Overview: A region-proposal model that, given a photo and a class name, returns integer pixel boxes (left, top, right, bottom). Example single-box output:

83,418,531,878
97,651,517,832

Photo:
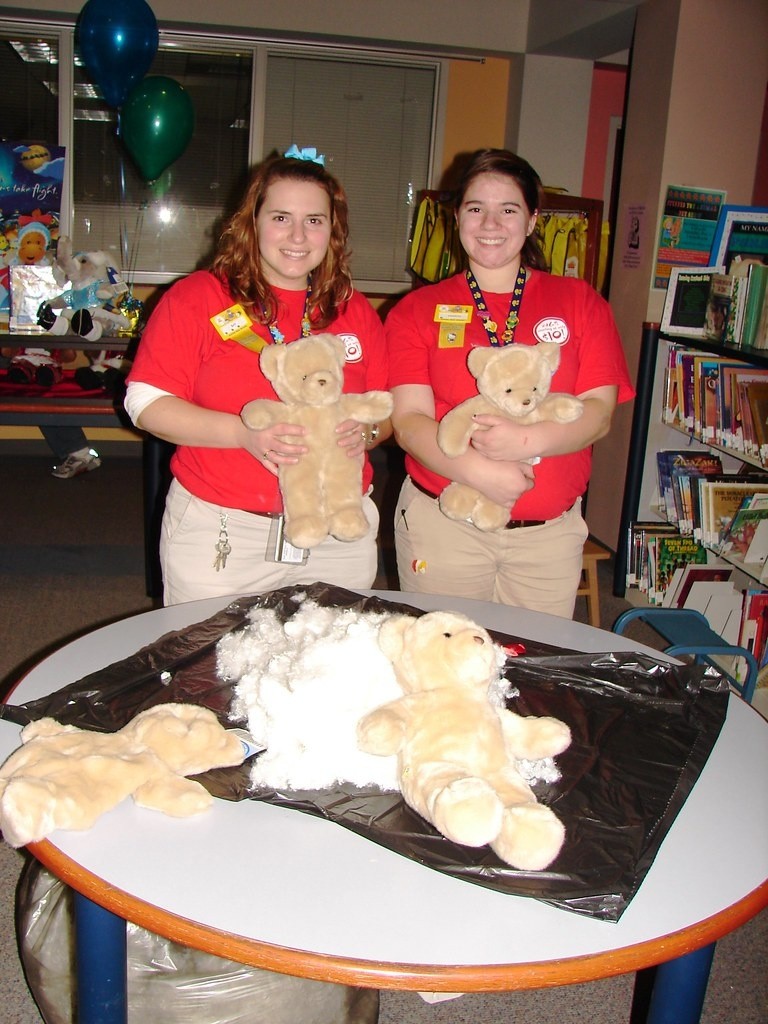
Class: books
650,185,768,355
657,447,767,586
660,343,768,468
624,521,768,692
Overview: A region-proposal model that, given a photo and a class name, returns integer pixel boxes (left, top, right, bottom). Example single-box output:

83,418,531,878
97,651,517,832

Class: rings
362,432,366,440
264,450,275,460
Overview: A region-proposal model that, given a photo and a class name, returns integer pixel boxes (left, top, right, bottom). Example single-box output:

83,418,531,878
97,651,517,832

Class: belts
410,474,577,531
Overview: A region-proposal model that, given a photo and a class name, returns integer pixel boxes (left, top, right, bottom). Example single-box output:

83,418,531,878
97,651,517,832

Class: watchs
372,423,380,442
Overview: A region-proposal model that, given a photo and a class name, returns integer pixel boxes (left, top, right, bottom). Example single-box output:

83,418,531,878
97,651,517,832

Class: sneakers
51,448,101,478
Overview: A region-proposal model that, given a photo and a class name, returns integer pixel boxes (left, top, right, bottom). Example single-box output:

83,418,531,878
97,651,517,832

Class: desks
0,587,767,1021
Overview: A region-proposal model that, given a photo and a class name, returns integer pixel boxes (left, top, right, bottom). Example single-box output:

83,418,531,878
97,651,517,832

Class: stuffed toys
240,329,370,547
358,610,572,872
435,340,584,532
42,232,131,342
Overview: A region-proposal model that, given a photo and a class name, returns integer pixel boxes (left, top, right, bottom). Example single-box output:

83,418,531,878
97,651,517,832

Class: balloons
73,0,159,110
123,76,193,184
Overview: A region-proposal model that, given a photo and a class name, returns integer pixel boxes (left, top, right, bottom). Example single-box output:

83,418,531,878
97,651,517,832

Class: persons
41,424,102,478
121,144,394,615
384,147,638,621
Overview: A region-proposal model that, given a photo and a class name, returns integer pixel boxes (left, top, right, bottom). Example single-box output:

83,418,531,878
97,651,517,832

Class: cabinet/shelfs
609,320,767,719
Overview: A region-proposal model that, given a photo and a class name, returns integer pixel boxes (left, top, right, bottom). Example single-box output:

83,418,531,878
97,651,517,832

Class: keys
212,542,232,573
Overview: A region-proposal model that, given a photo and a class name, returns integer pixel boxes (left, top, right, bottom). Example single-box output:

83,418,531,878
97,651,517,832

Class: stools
612,607,756,704
572,536,611,629
